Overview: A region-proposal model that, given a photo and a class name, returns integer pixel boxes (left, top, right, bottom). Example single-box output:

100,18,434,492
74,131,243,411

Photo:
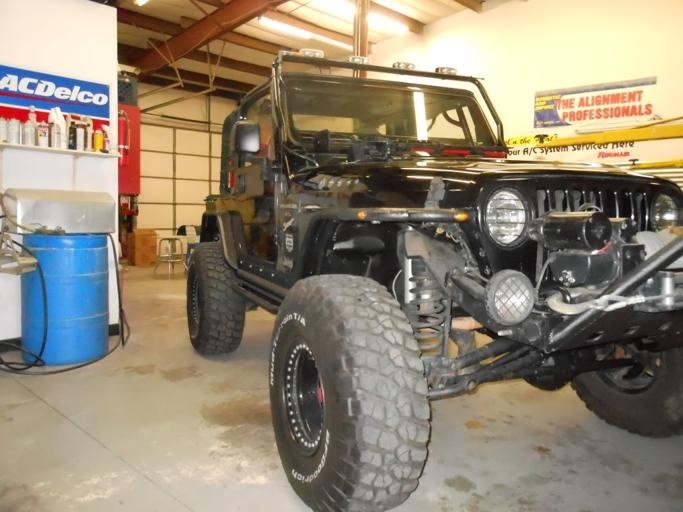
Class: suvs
186,46,683,512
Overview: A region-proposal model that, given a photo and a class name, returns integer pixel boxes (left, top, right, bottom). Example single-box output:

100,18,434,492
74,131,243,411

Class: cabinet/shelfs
0,96,122,160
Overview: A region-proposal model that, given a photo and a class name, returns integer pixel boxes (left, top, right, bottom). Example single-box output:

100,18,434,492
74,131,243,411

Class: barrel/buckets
20,233,110,365
48,107,67,150
